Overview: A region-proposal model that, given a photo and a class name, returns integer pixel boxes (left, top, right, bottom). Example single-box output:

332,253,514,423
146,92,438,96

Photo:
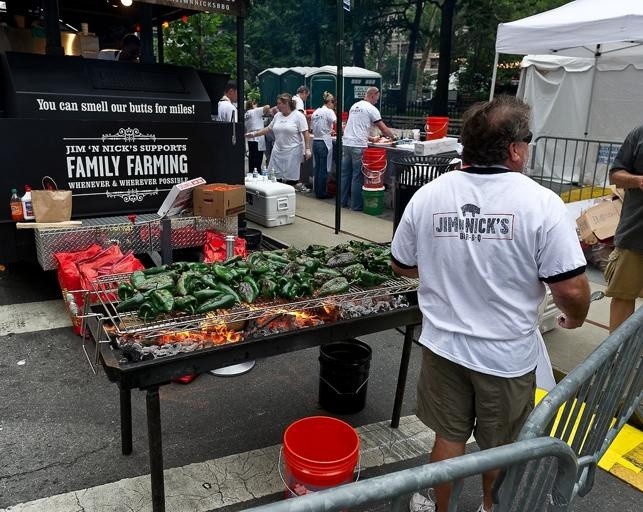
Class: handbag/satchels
30,174,74,224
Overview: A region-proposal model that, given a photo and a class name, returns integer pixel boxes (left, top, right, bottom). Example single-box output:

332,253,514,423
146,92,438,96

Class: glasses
517,130,532,144
375,89,380,94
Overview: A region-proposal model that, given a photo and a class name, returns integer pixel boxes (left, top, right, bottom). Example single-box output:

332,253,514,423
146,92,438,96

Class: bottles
252,166,276,182
9,188,23,221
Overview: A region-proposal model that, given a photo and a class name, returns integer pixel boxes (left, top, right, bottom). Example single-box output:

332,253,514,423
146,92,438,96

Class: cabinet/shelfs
79,294,421,511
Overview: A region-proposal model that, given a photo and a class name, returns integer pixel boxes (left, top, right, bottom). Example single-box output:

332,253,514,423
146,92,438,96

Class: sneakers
409,491,437,512
476,502,493,512
294,183,310,193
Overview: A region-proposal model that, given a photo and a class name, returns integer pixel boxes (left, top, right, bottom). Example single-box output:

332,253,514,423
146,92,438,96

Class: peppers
117,237,393,318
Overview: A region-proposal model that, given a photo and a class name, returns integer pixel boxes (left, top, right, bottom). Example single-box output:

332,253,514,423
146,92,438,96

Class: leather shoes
319,194,333,199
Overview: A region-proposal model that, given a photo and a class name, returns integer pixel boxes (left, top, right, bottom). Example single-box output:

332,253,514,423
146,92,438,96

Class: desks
329,132,459,189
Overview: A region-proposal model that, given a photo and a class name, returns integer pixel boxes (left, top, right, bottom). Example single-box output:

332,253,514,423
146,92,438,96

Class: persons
340,85,394,212
602,124,642,335
388,90,591,512
216,82,340,199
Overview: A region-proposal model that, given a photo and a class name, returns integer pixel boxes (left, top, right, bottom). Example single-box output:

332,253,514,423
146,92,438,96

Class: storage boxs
192,182,246,219
244,178,295,228
412,137,457,156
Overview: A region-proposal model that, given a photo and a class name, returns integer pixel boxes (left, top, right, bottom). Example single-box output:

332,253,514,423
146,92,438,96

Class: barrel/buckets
425,117,450,140
317,339,373,414
361,148,387,216
306,109,316,132
276,416,363,500
332,111,347,136
